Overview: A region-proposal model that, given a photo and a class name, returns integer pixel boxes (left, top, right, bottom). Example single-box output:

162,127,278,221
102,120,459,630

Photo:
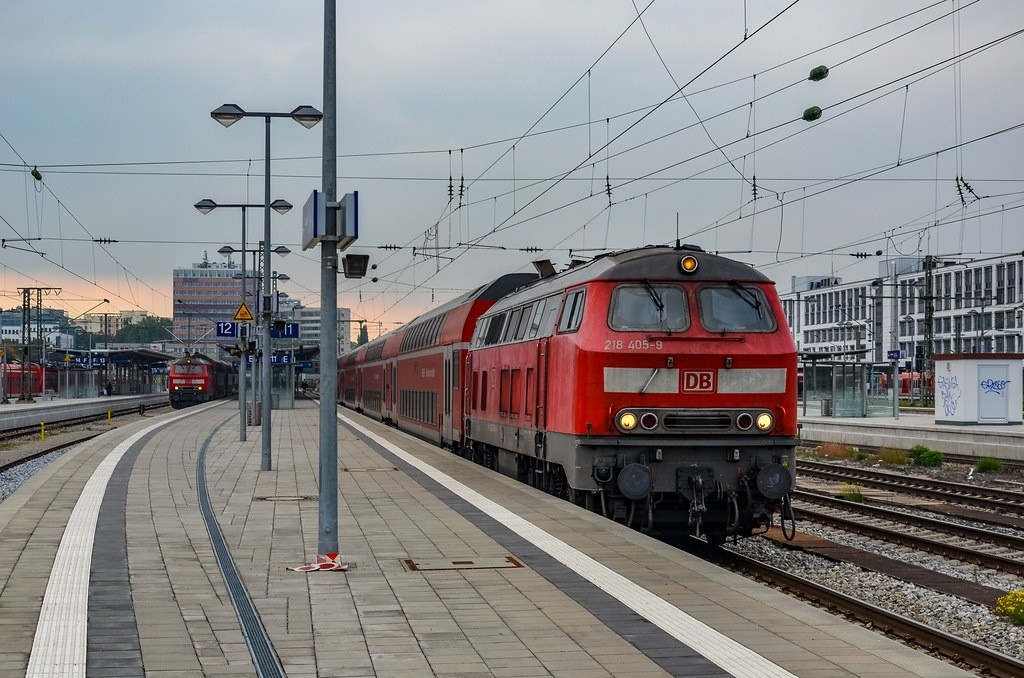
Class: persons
104,381,114,399
300,379,309,393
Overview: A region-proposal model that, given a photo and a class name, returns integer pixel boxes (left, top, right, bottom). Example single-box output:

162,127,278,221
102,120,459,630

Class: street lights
194,199,295,442
210,99,323,473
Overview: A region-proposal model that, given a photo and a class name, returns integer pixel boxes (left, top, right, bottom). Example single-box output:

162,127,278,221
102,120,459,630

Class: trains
338,237,800,548
168,350,240,409
0,362,58,396
